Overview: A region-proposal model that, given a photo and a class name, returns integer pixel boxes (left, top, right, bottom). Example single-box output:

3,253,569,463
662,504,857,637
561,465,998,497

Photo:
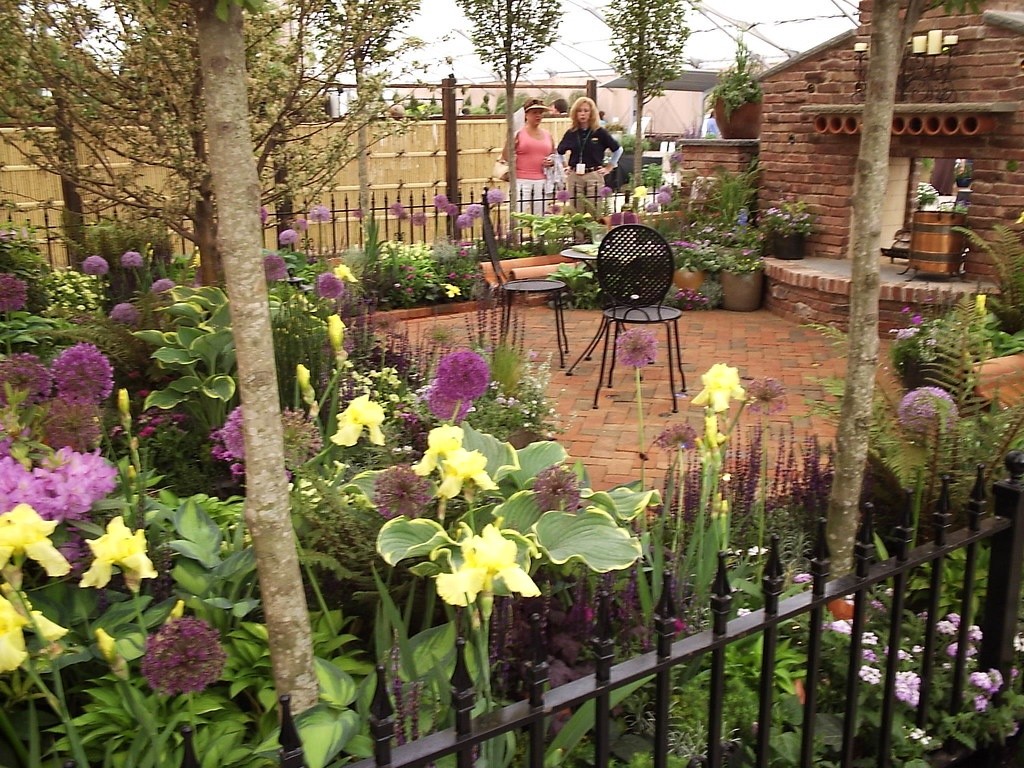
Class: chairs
593,223,688,413
481,201,570,369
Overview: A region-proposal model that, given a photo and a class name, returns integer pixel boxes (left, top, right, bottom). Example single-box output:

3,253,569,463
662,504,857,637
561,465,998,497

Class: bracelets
563,166,570,171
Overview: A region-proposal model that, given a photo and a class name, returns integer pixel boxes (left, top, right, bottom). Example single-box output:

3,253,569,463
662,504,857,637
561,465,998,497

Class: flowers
664,193,827,313
703,27,760,122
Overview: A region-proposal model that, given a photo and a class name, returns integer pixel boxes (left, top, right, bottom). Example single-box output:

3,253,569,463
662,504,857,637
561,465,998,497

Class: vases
673,265,708,294
715,97,759,140
719,270,760,313
956,177,971,187
774,230,807,259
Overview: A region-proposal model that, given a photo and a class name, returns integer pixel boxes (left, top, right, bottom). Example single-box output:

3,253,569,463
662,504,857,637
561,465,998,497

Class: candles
927,29,944,55
945,35,958,44
855,42,868,50
912,36,928,53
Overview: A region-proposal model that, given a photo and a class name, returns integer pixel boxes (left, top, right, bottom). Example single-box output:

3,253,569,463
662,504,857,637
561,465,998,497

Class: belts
569,165,603,174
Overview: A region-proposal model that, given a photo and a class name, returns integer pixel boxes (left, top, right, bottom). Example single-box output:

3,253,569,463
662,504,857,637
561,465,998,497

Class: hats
524,99,553,113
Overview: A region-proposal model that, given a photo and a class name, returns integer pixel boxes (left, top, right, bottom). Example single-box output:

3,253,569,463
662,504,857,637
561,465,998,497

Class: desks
561,245,659,383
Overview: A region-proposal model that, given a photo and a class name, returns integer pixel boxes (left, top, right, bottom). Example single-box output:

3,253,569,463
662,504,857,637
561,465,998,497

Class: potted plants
613,134,662,186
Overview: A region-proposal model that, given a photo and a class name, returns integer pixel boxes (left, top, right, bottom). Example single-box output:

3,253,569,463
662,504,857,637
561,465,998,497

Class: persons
548,98,568,114
389,105,405,121
706,109,721,139
598,110,606,126
555,97,624,244
498,97,556,234
